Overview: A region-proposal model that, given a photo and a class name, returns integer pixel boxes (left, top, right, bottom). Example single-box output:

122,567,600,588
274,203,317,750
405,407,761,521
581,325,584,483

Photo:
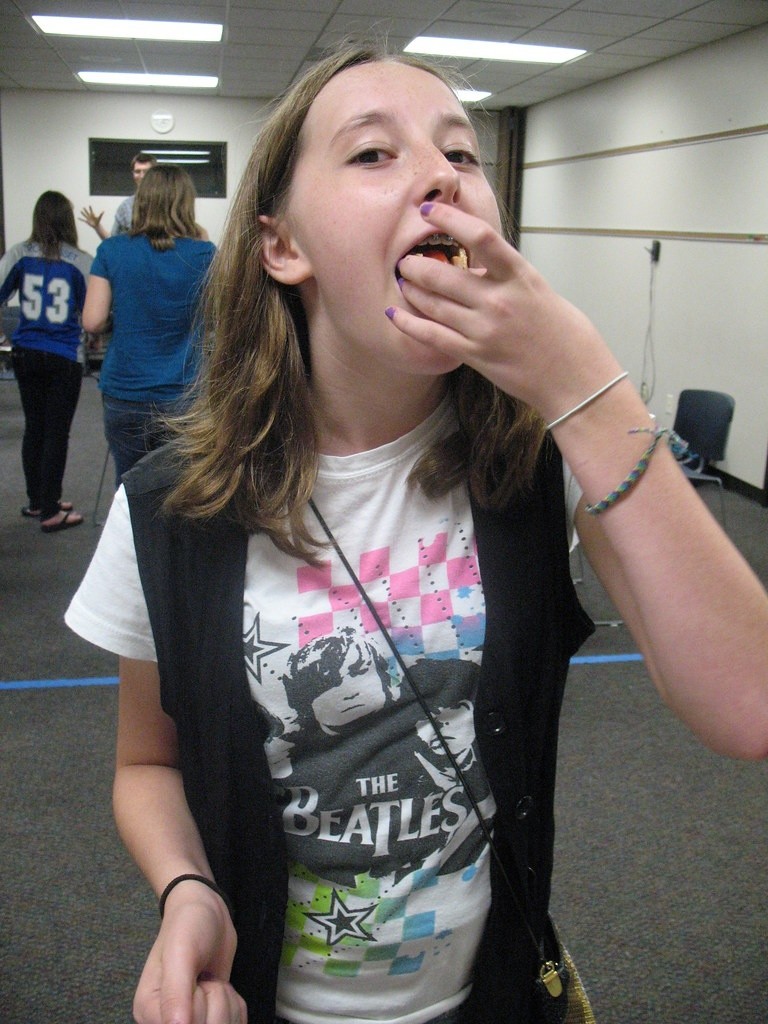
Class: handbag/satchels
534,914,592,1024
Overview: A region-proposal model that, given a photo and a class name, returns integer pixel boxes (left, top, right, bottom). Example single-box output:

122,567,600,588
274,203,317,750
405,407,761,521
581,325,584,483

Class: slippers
21,502,72,516
41,513,83,531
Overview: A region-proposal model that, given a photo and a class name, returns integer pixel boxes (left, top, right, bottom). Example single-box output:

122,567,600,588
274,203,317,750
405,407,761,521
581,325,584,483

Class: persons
59,41,767,1024
0,153,219,529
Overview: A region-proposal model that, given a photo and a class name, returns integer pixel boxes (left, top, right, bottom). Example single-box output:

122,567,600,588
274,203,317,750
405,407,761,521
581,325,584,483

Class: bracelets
158,873,230,919
543,370,628,428
585,423,689,515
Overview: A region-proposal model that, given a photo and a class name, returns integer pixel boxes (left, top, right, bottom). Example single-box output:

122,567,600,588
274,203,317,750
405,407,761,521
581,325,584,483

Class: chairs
672,389,735,524
560,456,624,626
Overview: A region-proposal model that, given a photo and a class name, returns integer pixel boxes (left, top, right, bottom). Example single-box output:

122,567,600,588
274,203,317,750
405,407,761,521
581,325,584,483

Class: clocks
151,109,175,134
